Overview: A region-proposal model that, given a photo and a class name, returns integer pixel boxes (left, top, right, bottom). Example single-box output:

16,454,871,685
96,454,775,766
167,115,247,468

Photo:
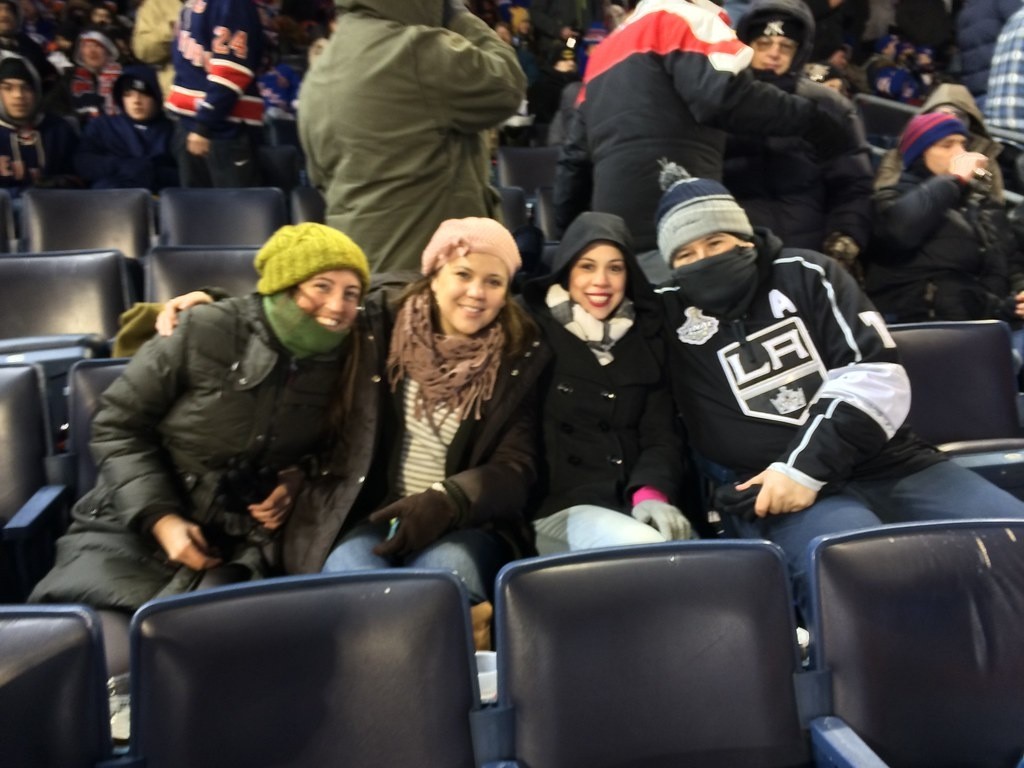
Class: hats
73,20,119,69
735,8,805,52
112,71,161,116
507,6,534,38
0,58,36,95
898,114,973,171
254,221,372,297
652,157,758,268
420,218,523,285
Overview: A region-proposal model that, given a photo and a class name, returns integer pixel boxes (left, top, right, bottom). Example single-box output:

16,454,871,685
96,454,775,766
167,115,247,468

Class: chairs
1,146,1024,768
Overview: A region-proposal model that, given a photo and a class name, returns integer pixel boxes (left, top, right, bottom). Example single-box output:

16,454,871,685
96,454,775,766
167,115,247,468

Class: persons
29,224,369,687
649,161,1024,655
537,207,697,557
468,0,544,144
862,0,1024,322
154,217,536,652
295,0,528,274
0,0,346,191
547,0,863,260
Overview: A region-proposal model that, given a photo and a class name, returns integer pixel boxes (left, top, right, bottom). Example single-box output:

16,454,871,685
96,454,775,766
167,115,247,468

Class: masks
672,245,758,304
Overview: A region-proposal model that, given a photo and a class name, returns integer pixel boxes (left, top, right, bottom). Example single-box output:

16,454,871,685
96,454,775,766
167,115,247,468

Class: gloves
367,490,459,569
633,487,693,543
